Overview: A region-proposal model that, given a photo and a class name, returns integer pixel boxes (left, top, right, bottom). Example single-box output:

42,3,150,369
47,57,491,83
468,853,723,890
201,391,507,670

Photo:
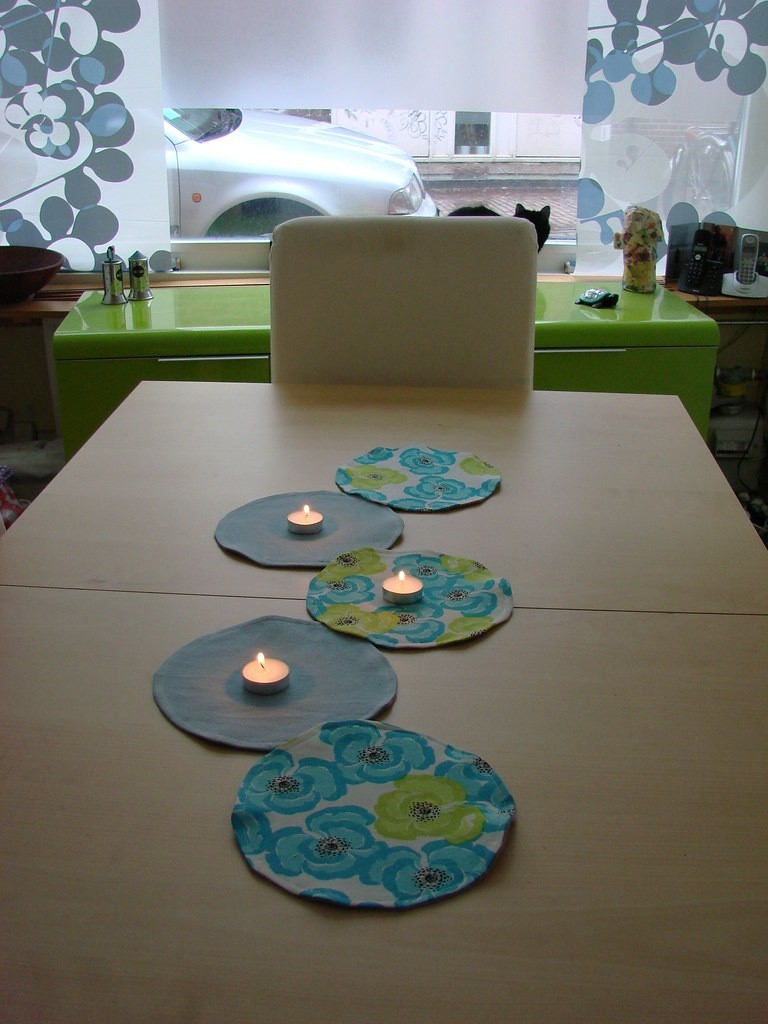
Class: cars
163,107,440,240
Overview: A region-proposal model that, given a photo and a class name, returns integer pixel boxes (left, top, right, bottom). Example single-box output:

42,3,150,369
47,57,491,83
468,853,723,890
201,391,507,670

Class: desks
1,380,768,1024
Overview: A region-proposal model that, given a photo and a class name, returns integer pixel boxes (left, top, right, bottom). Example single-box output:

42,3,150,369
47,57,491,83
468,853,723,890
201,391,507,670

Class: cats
447,204,551,253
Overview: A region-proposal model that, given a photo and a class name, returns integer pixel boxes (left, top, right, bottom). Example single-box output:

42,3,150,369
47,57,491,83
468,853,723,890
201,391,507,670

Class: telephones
722,234,768,298
677,229,725,295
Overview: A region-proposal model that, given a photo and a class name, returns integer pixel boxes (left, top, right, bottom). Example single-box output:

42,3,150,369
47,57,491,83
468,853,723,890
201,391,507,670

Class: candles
383,574,423,603
286,510,323,534
241,658,290,694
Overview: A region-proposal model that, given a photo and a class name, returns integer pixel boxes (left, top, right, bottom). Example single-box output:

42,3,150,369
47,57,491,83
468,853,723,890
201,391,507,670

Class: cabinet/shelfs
53,281,717,443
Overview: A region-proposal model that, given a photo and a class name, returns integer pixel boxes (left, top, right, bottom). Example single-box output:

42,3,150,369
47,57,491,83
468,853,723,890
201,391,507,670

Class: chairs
268,215,536,390
